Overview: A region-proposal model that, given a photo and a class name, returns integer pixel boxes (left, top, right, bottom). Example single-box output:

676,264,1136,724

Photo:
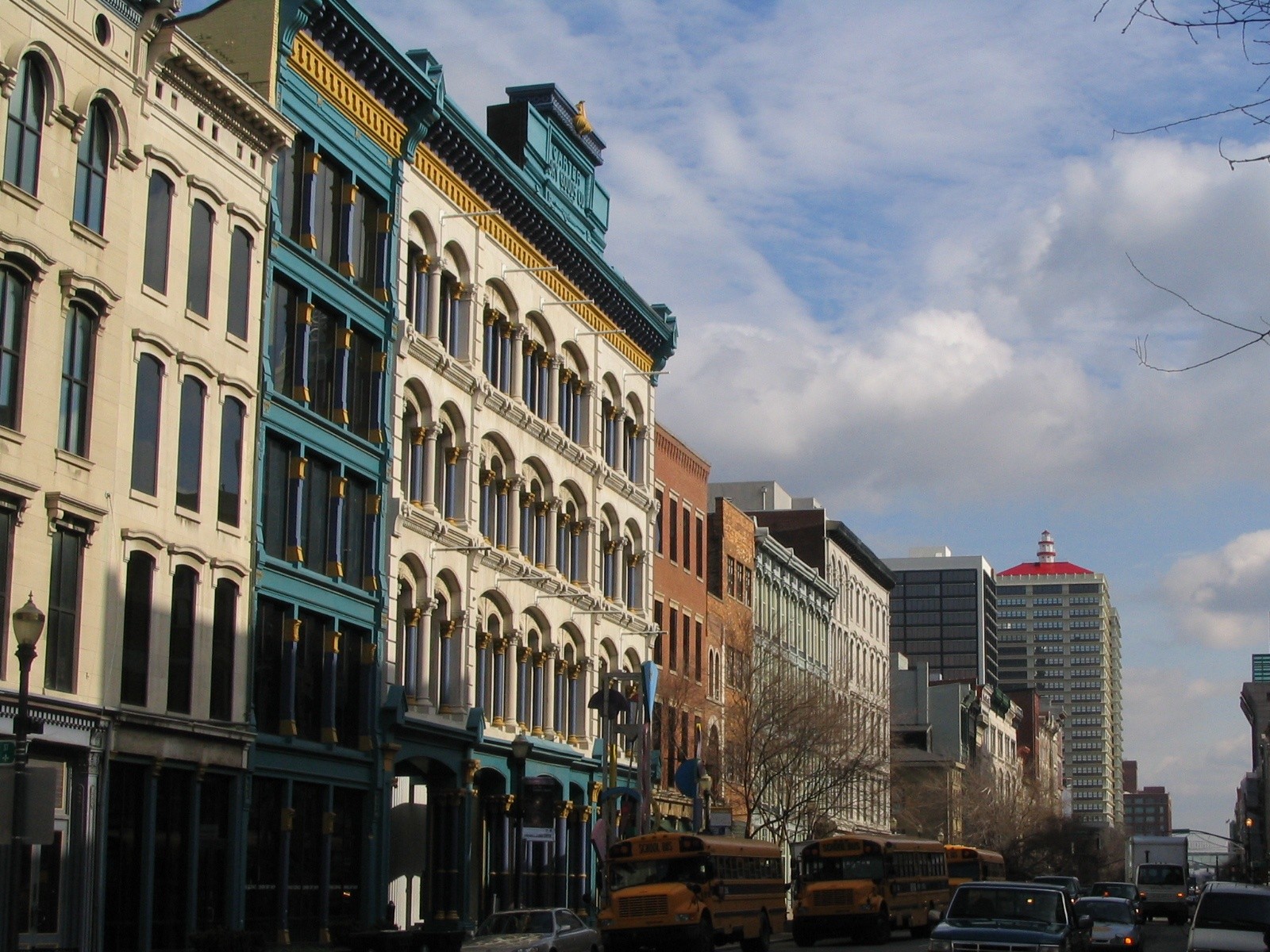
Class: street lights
4,597,46,952
696,774,713,831
509,735,531,916
1169,828,1248,881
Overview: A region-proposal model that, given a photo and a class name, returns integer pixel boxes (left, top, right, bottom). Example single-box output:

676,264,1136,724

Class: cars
1033,874,1088,907
1084,880,1146,919
1074,896,1144,952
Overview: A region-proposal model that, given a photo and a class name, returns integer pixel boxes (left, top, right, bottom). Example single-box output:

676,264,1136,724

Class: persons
1165,868,1180,885
1118,887,1130,898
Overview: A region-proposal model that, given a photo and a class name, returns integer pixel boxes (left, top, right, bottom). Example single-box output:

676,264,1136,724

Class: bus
595,831,786,951
791,833,950,945
944,846,1007,893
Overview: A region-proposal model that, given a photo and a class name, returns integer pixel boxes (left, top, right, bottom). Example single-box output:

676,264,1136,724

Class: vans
1188,881,1270,952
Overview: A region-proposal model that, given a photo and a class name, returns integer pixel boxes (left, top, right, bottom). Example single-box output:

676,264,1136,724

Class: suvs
927,880,1095,951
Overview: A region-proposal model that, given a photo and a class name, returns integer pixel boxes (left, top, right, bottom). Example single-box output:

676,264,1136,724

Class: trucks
1128,833,1199,924
